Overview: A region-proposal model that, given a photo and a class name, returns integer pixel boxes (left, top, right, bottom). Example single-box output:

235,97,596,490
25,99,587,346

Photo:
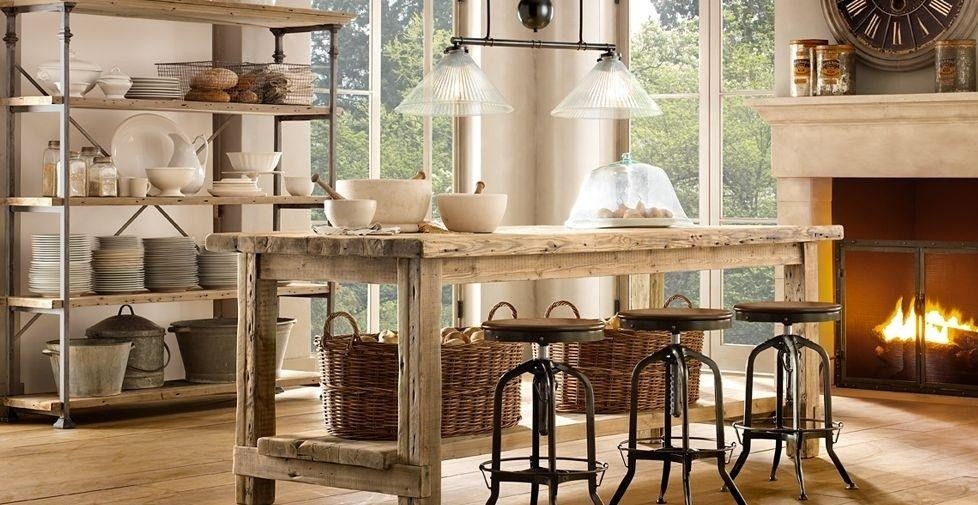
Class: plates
127,76,183,99
208,178,268,199
27,232,239,298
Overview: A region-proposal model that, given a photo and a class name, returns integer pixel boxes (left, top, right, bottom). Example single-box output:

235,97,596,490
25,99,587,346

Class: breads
184,67,258,102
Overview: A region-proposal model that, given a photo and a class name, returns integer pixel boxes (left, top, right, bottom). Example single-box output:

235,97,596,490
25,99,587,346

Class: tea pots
168,131,209,194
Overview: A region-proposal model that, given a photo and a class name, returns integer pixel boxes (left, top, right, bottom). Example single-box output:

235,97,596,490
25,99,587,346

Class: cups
120,176,151,197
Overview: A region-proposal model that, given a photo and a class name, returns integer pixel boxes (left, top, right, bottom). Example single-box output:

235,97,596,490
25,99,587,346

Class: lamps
393,0,663,120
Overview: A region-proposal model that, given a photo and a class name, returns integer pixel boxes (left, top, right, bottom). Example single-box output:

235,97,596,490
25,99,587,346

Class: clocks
814,1,977,72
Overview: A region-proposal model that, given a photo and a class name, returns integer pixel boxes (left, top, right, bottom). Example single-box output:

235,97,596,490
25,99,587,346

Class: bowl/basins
37,48,133,99
225,149,282,171
145,168,197,197
112,113,193,170
284,175,314,195
324,178,508,233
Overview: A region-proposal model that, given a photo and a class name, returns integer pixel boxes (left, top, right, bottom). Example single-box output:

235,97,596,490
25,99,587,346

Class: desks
203,223,847,505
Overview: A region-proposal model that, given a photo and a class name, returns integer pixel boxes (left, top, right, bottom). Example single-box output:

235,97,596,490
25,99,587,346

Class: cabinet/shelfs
0,0,358,430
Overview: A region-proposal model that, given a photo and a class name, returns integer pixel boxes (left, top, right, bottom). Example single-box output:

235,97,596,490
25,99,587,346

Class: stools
609,307,747,505
480,317,608,505
722,302,859,501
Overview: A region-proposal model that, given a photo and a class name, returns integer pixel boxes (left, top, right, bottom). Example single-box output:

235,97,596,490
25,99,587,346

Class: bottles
43,139,118,197
788,38,854,97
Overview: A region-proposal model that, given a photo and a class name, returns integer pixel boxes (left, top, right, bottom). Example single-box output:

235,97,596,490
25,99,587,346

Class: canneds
41,141,118,197
788,39,856,97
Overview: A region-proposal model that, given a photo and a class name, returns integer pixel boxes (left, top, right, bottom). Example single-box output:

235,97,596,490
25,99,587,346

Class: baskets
154,62,314,106
313,301,524,440
530,294,704,414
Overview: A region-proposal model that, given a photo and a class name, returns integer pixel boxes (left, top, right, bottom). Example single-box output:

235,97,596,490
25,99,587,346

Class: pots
42,304,298,399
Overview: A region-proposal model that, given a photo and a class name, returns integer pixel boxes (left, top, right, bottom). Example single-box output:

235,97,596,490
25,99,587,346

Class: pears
355,328,484,349
609,314,621,329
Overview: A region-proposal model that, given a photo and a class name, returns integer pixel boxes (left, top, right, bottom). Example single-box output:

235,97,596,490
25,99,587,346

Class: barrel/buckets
86,306,172,388
38,336,134,397
